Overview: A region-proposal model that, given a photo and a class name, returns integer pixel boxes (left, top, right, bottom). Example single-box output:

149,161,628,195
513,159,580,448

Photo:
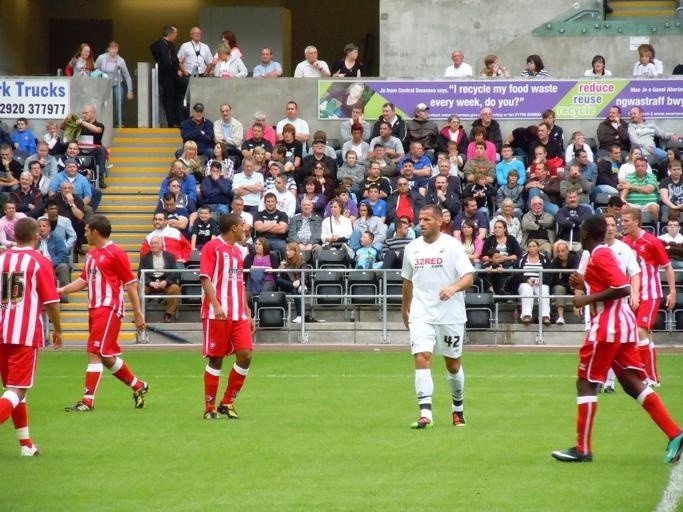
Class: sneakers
663,430,683,464
549,445,593,464
217,403,239,419
450,410,466,428
19,444,45,457
63,400,95,412
131,380,148,408
410,414,433,428
202,403,217,418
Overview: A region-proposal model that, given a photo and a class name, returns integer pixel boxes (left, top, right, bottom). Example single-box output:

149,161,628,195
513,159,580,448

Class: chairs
0,121,103,301
155,138,682,304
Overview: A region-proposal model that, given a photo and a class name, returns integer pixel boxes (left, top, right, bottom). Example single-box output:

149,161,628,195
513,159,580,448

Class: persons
213,30,248,78
295,46,332,78
633,44,664,77
619,206,676,389
521,55,549,77
1,103,107,303
199,214,254,419
332,43,362,78
94,41,133,129
480,55,509,76
150,25,185,127
584,56,611,77
399,202,476,430
445,50,472,76
54,214,148,412
572,215,642,395
177,27,213,83
550,214,682,463
139,102,683,324
0,218,63,455
253,48,283,78
65,43,94,77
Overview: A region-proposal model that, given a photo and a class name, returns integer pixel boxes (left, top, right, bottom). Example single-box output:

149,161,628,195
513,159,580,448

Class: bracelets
319,67,323,71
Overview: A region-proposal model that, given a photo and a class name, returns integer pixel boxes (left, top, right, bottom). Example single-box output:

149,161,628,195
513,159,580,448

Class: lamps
254,289,288,331
464,292,494,328
